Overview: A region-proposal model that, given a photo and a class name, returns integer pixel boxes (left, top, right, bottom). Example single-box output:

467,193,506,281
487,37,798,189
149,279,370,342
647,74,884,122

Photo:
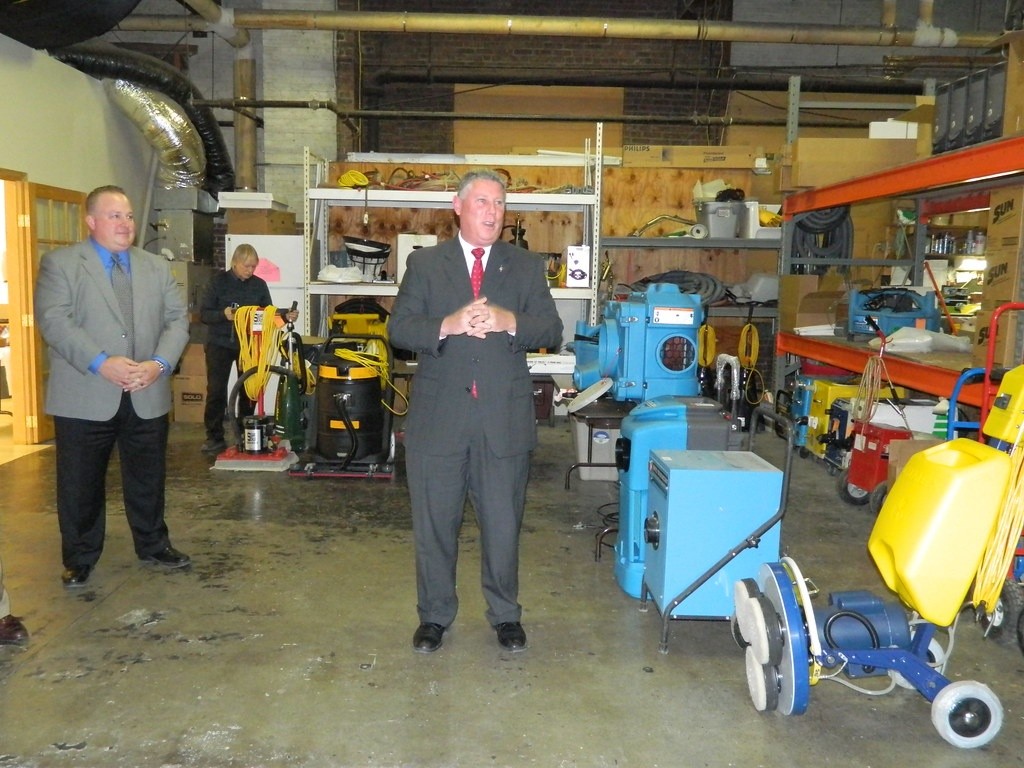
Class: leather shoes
497,621,527,651
62,557,93,586
138,546,190,567
412,622,446,653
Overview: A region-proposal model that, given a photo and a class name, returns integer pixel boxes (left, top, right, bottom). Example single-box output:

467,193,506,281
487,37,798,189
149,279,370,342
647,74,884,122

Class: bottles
924,229,987,255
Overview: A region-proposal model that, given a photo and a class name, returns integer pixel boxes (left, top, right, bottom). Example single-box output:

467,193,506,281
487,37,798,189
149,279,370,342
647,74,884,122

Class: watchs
156,360,164,373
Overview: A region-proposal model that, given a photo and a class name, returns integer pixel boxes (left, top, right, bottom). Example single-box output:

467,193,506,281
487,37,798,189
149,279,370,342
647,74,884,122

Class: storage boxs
983,30,1024,136
526,350,576,374
971,183,1024,365
849,398,958,442
695,201,743,239
569,414,622,481
562,245,590,288
781,274,847,334
218,192,289,212
396,231,438,283
887,439,945,494
226,208,297,235
173,343,207,423
773,104,937,193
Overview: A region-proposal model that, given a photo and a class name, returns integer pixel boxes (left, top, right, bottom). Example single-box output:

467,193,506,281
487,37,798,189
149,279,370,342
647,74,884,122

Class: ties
471,248,484,398
110,251,134,360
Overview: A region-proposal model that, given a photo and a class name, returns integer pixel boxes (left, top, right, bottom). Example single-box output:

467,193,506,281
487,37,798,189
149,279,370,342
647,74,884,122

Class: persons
388,169,565,653
199,244,299,451
36,185,192,586
0,559,29,644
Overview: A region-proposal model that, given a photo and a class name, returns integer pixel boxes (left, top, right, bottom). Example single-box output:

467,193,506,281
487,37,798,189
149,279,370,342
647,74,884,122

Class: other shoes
200,440,225,450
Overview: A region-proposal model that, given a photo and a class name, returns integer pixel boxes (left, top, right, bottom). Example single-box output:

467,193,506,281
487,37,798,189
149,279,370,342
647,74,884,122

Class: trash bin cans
568,376,637,482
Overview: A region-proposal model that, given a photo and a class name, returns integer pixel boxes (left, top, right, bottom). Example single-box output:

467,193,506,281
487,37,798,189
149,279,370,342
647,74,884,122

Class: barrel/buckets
243,415,269,455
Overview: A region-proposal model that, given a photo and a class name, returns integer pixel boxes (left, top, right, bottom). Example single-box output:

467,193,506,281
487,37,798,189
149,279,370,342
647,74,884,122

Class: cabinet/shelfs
304,121,604,382
598,236,780,318
884,223,988,321
767,74,1024,437
